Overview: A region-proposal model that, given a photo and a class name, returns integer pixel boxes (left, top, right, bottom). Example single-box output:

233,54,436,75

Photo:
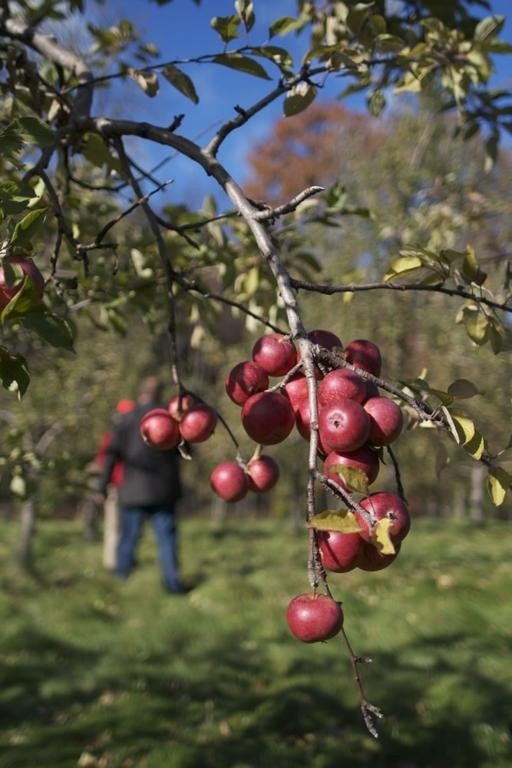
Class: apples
137,330,410,642
0,256,44,311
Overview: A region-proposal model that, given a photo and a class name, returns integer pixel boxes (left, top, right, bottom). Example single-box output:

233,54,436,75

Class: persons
88,375,189,593
96,397,141,572
